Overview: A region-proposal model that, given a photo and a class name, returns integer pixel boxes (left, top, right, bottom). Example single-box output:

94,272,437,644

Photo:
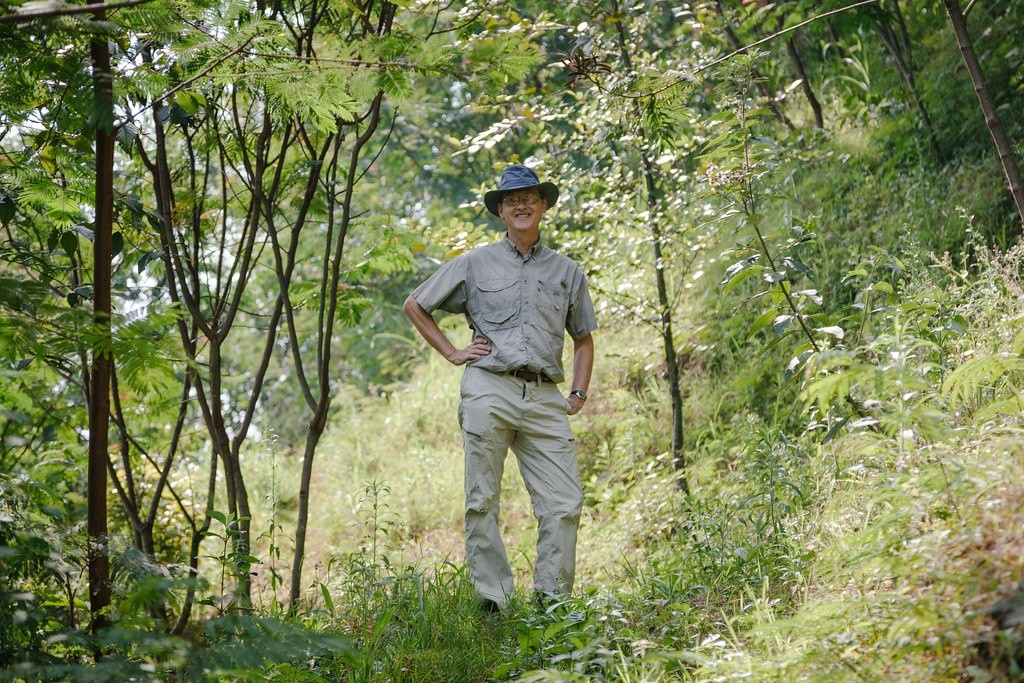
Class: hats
484,165,559,217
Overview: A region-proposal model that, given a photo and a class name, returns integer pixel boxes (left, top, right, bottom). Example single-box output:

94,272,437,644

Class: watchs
571,389,587,401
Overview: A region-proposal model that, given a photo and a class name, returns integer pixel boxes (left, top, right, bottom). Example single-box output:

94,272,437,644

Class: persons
402,165,597,615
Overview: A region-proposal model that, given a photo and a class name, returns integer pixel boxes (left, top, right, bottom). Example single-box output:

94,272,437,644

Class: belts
509,369,550,381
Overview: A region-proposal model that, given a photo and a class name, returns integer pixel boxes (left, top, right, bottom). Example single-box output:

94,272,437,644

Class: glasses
501,194,542,206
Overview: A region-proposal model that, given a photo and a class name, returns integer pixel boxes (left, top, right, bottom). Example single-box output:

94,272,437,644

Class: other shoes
479,601,501,616
